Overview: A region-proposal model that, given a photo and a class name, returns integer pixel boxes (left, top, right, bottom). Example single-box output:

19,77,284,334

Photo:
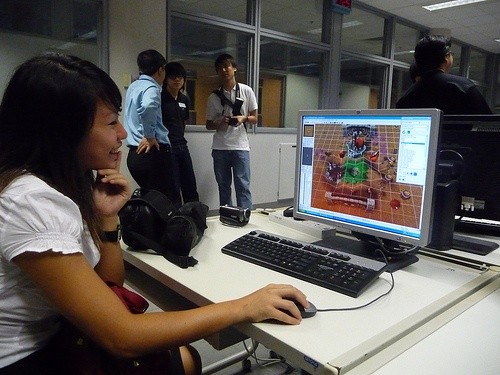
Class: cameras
219,204,250,226
226,97,245,125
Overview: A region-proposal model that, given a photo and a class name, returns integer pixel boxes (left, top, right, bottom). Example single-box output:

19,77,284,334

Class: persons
161,62,199,206
411,60,421,83
395,35,492,115
0,53,310,375
124,49,180,210
206,53,258,210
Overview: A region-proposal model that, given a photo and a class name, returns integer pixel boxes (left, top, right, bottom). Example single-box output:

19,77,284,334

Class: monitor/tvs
427,115,500,256
294,107,443,273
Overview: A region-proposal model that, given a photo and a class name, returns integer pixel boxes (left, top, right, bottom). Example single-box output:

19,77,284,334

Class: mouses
264,297,317,321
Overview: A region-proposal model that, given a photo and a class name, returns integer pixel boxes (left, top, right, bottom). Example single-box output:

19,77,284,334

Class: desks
120,206,500,375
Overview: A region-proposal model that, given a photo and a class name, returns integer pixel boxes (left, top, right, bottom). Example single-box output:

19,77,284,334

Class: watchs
102,229,121,242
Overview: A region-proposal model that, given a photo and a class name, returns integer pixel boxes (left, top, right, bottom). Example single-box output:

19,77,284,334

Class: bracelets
245,117,248,122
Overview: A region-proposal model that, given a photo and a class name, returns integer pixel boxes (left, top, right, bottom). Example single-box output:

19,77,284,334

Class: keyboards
454,218,500,237
221,230,387,298
